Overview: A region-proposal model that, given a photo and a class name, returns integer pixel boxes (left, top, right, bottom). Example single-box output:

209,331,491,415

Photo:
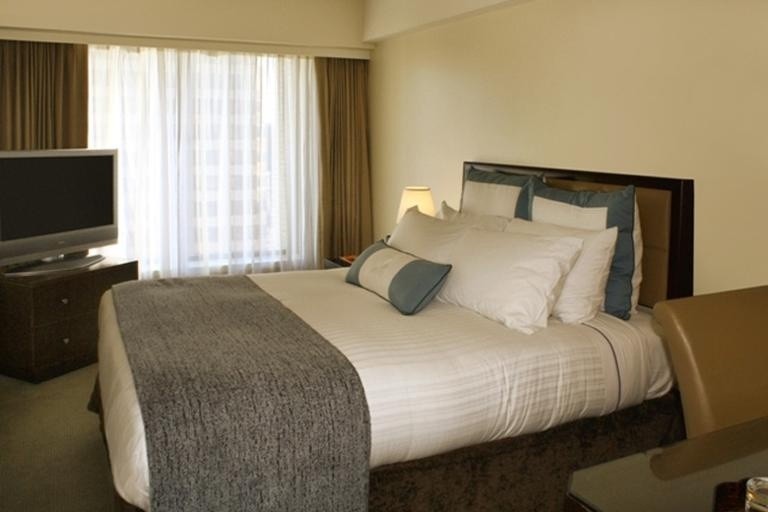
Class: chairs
651,284,768,436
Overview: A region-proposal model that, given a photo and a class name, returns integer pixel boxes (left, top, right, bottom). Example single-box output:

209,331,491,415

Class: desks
563,413,768,512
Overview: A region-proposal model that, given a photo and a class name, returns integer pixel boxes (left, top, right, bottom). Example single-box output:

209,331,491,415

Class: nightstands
322,254,359,269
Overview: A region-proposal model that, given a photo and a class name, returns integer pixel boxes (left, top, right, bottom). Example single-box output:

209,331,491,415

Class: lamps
396,185,435,222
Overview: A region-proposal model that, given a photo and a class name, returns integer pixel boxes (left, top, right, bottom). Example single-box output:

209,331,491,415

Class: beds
87,160,696,512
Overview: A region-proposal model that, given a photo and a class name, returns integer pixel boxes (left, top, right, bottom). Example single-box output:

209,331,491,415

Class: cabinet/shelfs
0,258,140,386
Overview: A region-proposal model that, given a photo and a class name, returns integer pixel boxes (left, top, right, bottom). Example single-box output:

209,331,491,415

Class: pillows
458,165,538,220
526,174,645,321
502,216,620,327
384,205,482,268
343,237,453,312
439,200,512,233
435,227,586,336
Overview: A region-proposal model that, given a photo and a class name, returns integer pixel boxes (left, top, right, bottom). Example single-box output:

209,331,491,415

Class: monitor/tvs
0,148,119,277
